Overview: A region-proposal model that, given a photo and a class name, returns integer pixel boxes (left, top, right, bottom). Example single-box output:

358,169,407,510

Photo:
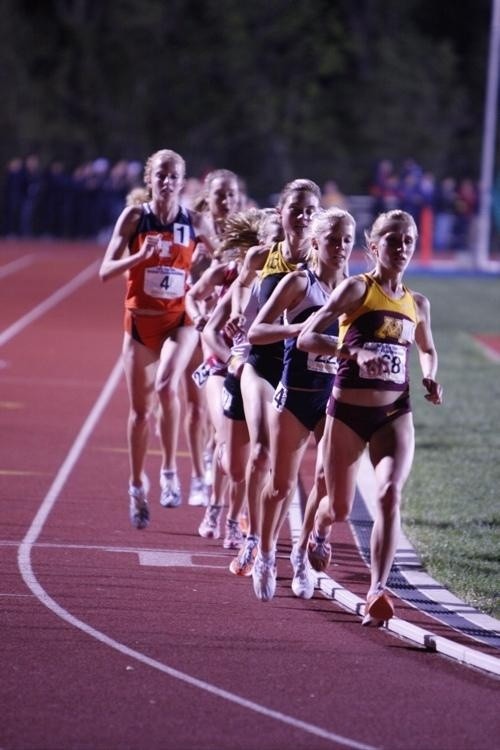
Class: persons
98,144,319,579
295,209,445,628
2,150,500,261
247,207,358,596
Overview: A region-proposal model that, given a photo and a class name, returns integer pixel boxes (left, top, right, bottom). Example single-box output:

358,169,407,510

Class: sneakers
126,470,151,530
197,501,224,540
250,544,278,602
158,465,184,508
307,523,334,574
288,543,319,600
359,589,395,630
229,534,259,578
222,514,246,552
187,475,212,508
202,450,216,486
